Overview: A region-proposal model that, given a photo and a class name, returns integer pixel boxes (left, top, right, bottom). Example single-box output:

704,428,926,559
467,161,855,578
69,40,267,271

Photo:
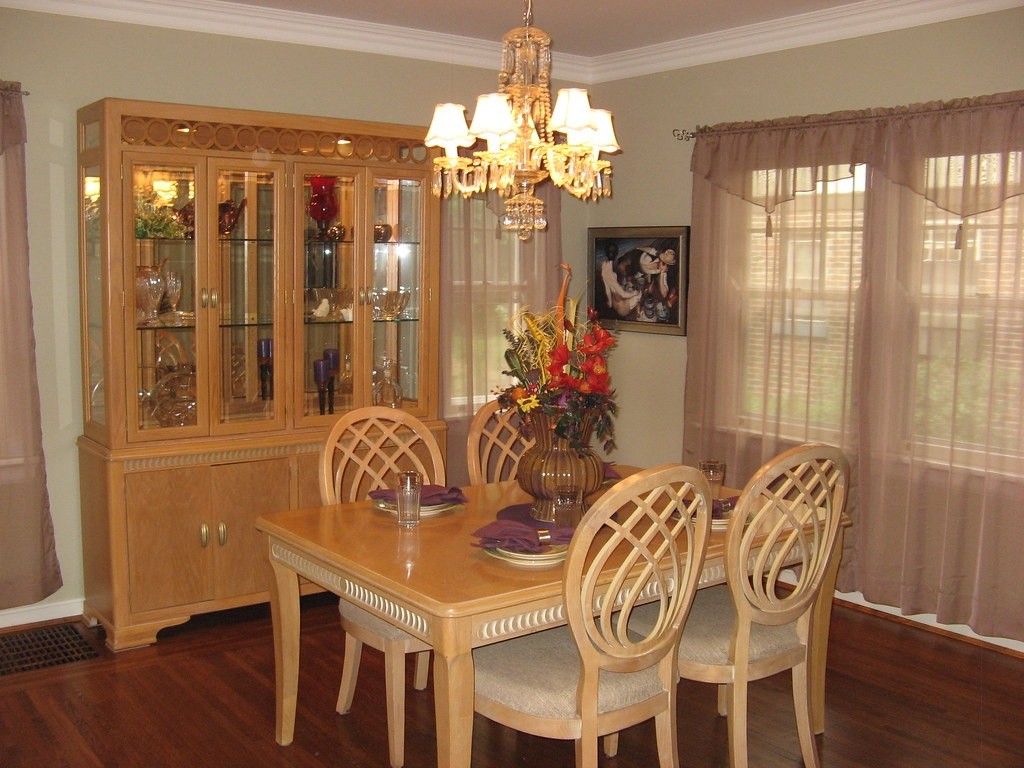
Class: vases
516,405,605,524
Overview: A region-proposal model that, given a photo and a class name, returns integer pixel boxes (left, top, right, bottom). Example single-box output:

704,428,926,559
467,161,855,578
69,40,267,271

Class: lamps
424,0,621,241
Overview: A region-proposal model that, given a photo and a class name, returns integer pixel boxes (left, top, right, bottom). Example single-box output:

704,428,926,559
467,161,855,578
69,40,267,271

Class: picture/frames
586,225,690,336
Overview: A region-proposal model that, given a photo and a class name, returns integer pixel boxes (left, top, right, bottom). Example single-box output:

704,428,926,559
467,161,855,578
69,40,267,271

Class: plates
373,499,459,516
482,544,569,567
670,511,751,530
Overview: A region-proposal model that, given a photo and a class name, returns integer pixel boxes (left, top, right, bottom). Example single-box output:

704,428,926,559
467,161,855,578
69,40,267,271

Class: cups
699,459,725,499
553,485,583,527
395,470,424,527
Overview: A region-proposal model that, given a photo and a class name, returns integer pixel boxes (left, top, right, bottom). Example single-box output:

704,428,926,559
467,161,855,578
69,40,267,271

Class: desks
254,464,854,768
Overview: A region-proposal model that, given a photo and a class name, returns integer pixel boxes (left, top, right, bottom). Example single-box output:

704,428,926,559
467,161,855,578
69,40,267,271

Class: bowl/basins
313,286,353,315
371,288,410,321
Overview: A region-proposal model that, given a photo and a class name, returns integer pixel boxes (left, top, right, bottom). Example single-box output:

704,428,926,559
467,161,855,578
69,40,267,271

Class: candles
258,340,271,358
314,349,338,382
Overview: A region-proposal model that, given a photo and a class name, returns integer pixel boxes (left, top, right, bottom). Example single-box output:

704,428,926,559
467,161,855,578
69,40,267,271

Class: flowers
490,263,619,460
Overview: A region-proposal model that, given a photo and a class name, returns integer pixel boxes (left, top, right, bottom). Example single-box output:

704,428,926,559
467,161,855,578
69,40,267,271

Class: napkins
470,519,574,554
671,495,739,519
368,484,470,506
513,461,623,482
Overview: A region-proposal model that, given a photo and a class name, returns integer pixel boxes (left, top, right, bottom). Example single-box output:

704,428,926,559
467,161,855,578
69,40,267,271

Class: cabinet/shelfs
74,97,450,654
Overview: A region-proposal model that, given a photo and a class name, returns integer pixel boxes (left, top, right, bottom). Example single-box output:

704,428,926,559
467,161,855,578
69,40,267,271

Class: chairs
318,399,538,768
471,442,852,768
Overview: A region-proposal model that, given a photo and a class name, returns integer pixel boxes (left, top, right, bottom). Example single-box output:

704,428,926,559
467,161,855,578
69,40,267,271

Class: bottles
324,349,340,414
371,358,403,409
313,359,328,415
165,272,182,311
306,175,339,234
257,339,274,401
134,265,165,322
339,353,354,410
